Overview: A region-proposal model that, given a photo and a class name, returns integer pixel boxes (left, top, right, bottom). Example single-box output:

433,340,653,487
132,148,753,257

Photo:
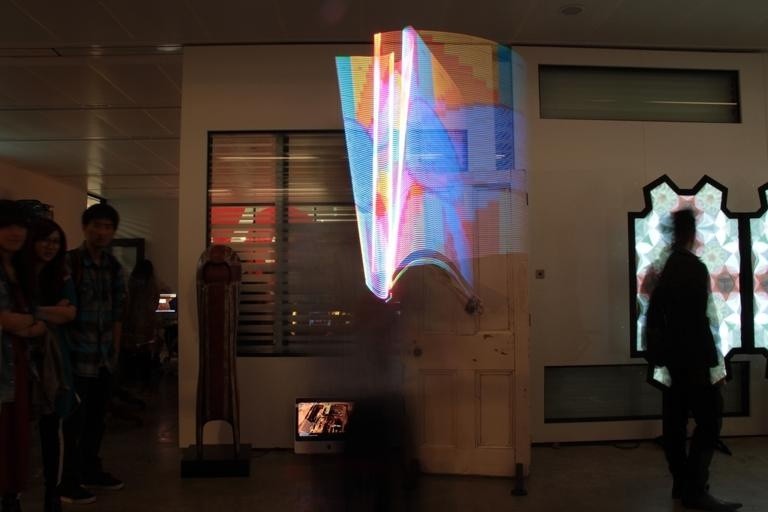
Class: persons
641,209,743,512
1,197,160,512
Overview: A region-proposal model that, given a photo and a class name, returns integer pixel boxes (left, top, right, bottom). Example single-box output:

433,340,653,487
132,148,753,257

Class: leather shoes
674,491,743,512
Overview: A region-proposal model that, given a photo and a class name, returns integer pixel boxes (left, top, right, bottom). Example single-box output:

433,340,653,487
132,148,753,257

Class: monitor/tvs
293,396,354,456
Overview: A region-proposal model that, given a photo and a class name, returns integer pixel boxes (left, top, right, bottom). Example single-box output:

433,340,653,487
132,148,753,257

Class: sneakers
60,487,97,504
81,472,125,490
